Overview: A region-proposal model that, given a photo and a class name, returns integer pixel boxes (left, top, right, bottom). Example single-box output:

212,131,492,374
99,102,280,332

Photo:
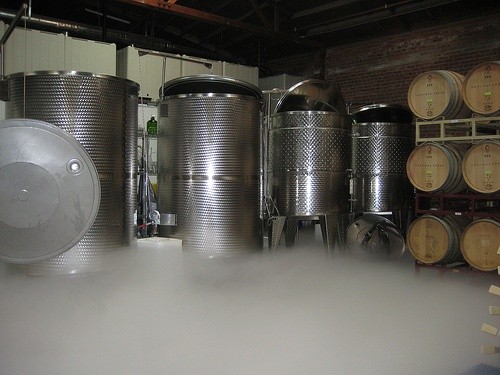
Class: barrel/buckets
156,75,263,255
264,94,346,221
4,70,140,277
459,217,500,272
405,142,466,193
406,213,463,265
462,61,499,117
347,103,406,215
406,70,470,121
460,137,500,194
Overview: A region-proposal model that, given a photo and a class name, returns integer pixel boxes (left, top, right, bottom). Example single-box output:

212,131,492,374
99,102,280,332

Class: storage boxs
138,96,152,104
